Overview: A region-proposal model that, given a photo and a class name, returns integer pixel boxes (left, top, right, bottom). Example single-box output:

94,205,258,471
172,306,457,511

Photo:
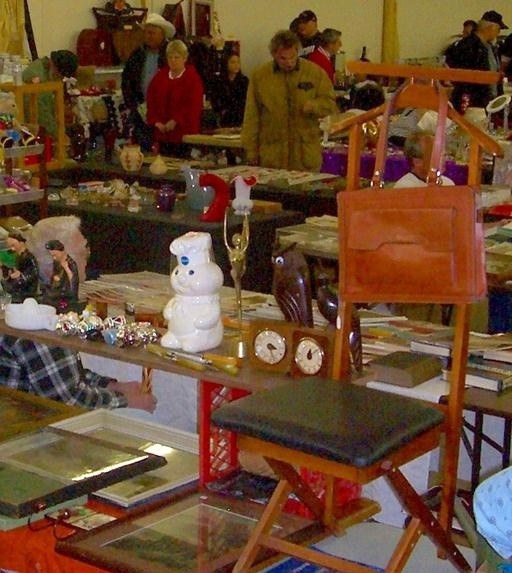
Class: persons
396,134,454,187
447,19,477,70
122,13,177,152
144,39,204,159
6,233,39,300
308,29,342,87
24,49,78,132
210,51,250,167
342,57,385,111
296,10,322,59
498,33,512,81
241,30,337,173
43,239,80,297
1,332,158,414
447,10,509,110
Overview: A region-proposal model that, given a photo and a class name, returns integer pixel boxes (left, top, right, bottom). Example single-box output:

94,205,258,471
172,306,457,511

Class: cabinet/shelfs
1,138,49,222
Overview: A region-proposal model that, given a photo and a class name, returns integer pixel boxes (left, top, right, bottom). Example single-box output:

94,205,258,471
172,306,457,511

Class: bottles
11,58,23,87
149,155,168,175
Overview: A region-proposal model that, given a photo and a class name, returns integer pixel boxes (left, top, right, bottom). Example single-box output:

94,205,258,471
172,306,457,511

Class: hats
297,9,317,24
136,12,175,40
481,10,509,29
50,49,77,78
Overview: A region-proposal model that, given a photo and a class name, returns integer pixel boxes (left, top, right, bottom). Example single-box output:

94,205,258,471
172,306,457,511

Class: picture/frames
0,407,382,573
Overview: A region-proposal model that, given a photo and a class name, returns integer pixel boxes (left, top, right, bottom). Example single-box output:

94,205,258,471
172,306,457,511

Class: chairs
202,57,507,573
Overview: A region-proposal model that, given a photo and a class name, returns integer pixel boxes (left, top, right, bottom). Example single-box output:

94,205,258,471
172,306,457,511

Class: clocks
244,316,351,384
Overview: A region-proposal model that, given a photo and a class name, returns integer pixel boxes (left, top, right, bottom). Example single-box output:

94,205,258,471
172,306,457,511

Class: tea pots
119,143,145,171
